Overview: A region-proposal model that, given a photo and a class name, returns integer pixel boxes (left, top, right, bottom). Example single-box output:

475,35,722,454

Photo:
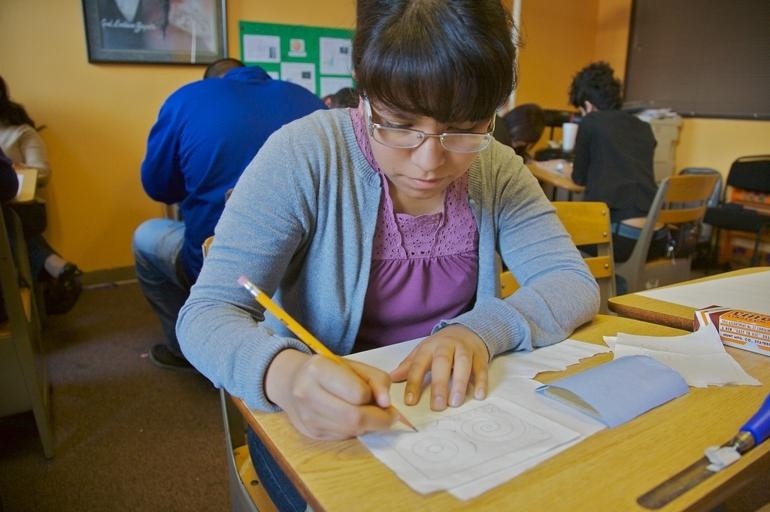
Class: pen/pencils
732,395,770,452
237,277,419,432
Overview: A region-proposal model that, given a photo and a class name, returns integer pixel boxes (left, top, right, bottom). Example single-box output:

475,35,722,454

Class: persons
566,60,672,268
331,86,359,110
132,56,376,374
175,2,604,512
0,75,82,295
0,147,21,331
322,94,331,108
491,103,544,167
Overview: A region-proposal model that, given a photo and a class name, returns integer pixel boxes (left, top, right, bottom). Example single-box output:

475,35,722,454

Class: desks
4,167,56,458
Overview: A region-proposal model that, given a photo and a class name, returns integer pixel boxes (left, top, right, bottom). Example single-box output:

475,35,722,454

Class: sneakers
150,343,197,372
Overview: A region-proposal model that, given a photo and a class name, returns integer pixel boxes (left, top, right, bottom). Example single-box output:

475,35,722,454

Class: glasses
363,69,498,153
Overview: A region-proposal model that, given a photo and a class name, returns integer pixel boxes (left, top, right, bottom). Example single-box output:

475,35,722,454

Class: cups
561,122,577,150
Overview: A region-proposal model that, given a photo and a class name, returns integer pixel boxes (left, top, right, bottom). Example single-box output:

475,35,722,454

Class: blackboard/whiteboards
622,0,770,119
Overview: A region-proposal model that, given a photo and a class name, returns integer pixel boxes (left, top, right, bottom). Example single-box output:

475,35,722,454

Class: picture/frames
81,1,228,66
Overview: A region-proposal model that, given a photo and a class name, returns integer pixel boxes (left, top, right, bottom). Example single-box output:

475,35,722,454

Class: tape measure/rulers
637,435,753,509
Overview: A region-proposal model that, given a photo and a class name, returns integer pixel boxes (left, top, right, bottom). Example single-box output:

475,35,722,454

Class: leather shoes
55,263,83,288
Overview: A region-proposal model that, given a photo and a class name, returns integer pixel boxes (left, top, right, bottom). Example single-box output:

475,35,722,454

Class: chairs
137,106,768,512
